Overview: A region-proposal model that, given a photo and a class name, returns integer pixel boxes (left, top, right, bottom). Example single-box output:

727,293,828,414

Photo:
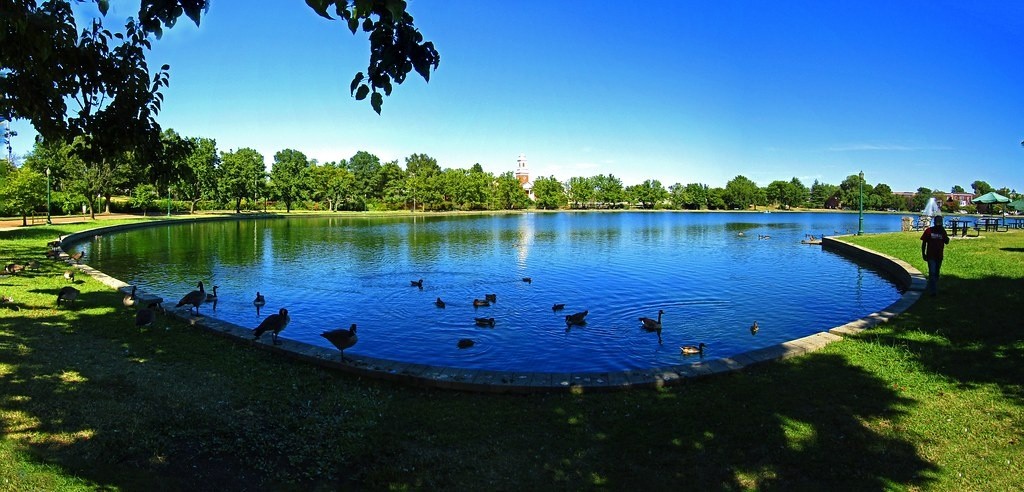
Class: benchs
947,222,1024,237
118,285,164,320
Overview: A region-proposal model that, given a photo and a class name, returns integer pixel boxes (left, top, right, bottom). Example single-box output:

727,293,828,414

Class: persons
921,216,949,291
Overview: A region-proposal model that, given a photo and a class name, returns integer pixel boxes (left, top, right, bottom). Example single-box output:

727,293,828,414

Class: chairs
909,215,932,231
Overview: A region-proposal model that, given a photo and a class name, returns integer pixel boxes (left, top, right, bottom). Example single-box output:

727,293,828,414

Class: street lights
46,167,51,226
168,188,171,216
858,170,864,236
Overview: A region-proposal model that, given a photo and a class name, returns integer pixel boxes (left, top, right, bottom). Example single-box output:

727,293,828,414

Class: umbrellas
971,192,1012,223
923,198,942,217
1007,198,1024,211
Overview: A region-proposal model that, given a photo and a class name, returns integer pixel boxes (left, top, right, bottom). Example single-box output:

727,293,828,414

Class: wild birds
254,292,265,307
475,317,495,327
750,321,759,332
679,343,706,354
639,310,665,329
206,286,219,302
458,338,474,349
552,303,565,310
522,277,532,284
437,297,445,308
135,304,156,331
0,241,85,307
251,308,290,342
122,286,139,307
565,310,589,324
320,324,357,356
474,299,489,306
411,279,423,286
175,281,207,316
486,293,496,301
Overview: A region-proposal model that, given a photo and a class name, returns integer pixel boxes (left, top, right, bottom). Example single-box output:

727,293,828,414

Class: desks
981,218,1001,232
1014,218,1024,228
949,220,972,236
